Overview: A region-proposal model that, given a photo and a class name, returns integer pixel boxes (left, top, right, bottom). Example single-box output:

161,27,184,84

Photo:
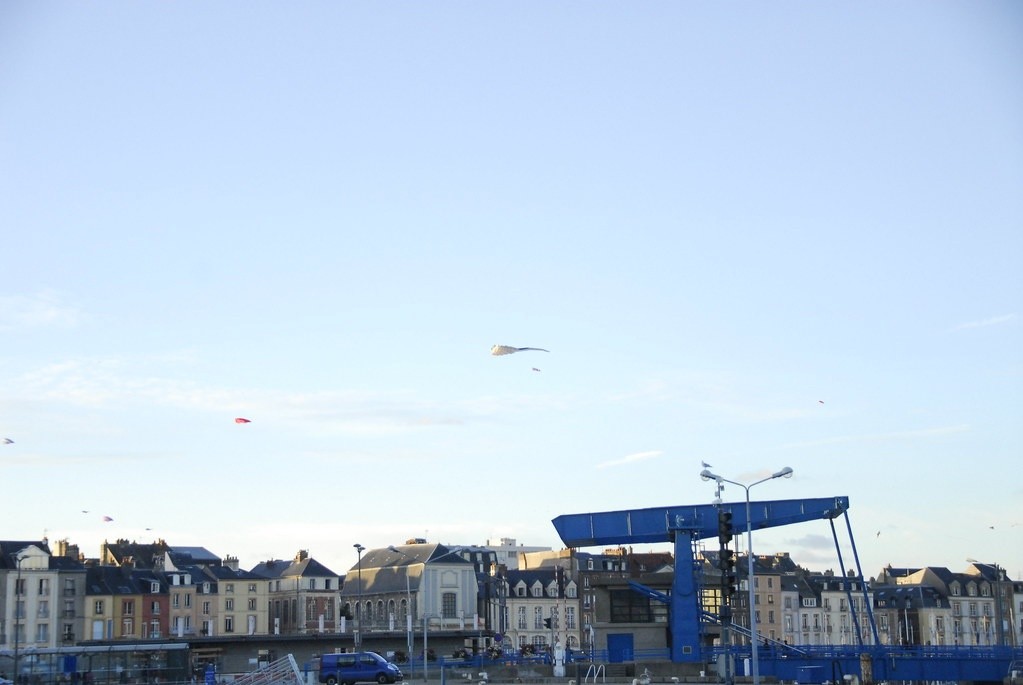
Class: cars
0,678,13,685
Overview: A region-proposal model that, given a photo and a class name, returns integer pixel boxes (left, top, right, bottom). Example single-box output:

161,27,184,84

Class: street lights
8,552,32,685
699,464,794,685
387,543,463,683
353,542,367,653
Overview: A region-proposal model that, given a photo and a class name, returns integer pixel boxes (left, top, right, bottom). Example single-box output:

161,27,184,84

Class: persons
764,638,789,649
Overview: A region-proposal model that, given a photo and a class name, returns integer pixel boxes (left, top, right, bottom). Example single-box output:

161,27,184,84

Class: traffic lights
719,549,734,571
721,575,736,598
543,618,552,628
717,509,733,545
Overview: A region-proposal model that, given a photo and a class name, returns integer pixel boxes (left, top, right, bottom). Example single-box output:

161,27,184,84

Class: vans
318,652,405,685
